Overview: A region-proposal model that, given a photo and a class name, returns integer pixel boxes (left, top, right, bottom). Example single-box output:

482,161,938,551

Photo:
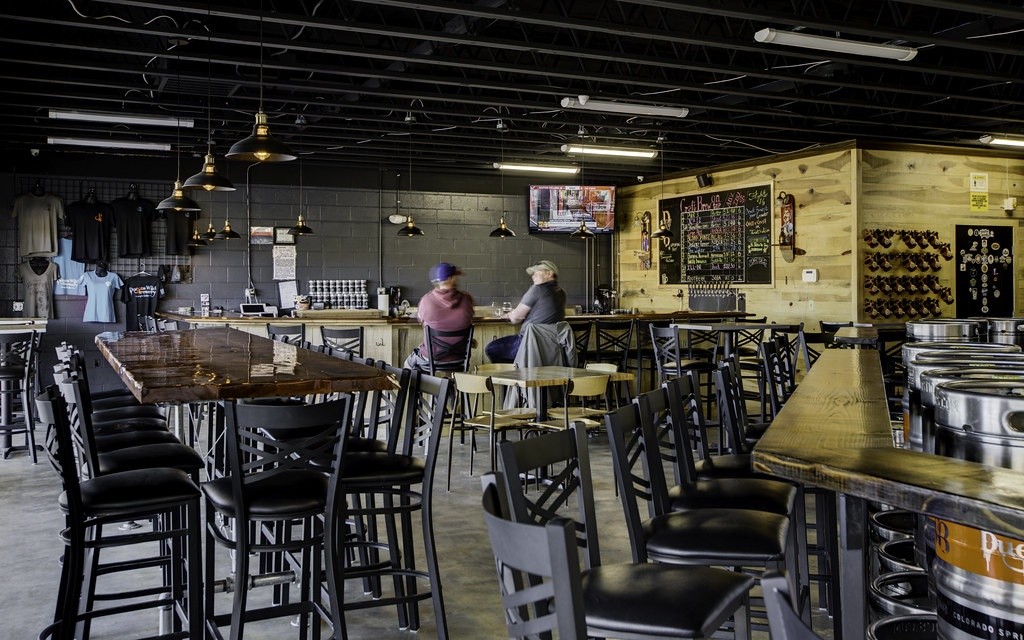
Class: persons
485,260,566,364
404,263,472,411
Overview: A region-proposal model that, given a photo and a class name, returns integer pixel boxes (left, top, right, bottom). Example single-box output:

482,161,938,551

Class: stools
0,318,996,640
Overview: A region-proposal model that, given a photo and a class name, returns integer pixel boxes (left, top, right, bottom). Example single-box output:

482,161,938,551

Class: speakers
696,172,710,188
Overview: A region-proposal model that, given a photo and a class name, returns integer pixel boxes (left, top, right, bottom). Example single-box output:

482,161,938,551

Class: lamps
215,145,240,240
287,154,314,236
493,161,581,175
754,29,918,62
561,97,690,117
48,109,196,129
200,191,217,240
489,121,516,239
563,145,658,158
398,119,425,237
183,0,234,191
187,192,208,246
979,135,1024,148
155,1,200,210
225,1,297,163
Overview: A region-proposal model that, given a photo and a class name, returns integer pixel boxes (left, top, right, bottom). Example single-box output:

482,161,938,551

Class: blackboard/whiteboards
656,180,779,290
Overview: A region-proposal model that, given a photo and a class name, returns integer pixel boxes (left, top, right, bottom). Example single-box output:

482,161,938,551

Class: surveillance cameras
637,176,643,182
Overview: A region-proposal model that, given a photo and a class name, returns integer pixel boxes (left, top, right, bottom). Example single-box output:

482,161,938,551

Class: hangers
129,263,153,277
28,178,150,201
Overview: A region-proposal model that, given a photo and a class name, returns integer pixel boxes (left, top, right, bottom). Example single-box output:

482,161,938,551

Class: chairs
525,375,618,507
448,371,539,494
473,364,539,473
550,364,620,483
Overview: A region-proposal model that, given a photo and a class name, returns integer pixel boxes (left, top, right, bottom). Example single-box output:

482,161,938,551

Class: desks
670,321,801,452
751,322,1024,639
482,364,636,488
94,326,401,640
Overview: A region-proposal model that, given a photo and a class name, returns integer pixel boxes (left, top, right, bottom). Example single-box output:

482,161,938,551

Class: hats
429,262,461,282
526,259,559,279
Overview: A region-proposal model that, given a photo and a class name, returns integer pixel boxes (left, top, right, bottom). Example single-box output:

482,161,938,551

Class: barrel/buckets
896,315,1024,640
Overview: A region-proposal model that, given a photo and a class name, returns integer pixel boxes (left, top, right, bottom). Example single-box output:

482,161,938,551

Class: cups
495,309,503,316
502,301,512,314
491,302,500,318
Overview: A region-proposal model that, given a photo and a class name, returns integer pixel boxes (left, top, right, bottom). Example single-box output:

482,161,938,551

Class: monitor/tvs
527,183,618,234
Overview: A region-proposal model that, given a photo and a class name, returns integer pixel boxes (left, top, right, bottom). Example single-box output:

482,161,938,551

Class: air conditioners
48,136,172,152
